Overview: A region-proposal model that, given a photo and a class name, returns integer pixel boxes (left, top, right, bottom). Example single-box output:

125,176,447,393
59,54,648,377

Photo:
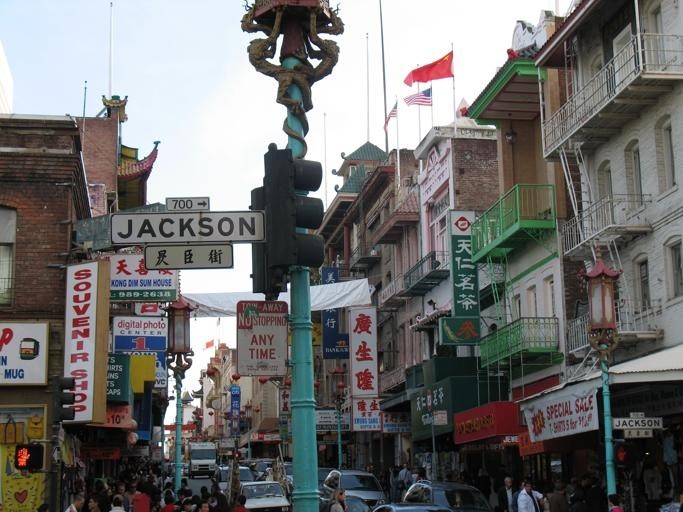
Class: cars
168,462,189,476
210,457,293,512
319,468,386,511
370,500,456,512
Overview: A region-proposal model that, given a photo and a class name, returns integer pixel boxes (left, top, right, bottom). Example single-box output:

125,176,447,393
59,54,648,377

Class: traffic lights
13,441,44,471
49,373,75,424
262,143,325,269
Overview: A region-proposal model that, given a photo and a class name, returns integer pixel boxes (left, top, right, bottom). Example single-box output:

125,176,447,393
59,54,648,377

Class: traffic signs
109,195,267,269
612,412,663,439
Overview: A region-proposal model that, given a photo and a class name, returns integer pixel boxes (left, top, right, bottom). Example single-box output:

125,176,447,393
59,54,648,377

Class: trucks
187,439,218,479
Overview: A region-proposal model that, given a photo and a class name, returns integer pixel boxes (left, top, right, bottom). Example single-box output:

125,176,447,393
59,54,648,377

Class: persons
546,462,675,511
325,486,348,511
365,463,427,502
444,469,544,511
61,461,291,512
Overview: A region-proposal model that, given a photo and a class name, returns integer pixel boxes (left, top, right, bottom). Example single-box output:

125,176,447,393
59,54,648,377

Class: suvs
401,477,493,512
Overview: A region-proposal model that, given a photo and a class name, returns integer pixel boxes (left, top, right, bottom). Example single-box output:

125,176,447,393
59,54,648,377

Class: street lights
326,363,346,470
160,293,199,498
574,238,623,510
157,385,169,478
242,398,255,460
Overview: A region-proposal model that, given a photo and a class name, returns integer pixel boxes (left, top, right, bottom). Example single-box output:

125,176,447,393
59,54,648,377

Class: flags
403,87,431,107
383,102,397,132
205,340,214,348
403,50,455,87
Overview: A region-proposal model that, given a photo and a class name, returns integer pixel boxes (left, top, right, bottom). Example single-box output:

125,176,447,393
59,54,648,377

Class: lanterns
258,377,267,383
191,404,260,420
314,381,319,387
231,374,240,381
206,368,214,375
336,383,344,387
286,379,291,385
